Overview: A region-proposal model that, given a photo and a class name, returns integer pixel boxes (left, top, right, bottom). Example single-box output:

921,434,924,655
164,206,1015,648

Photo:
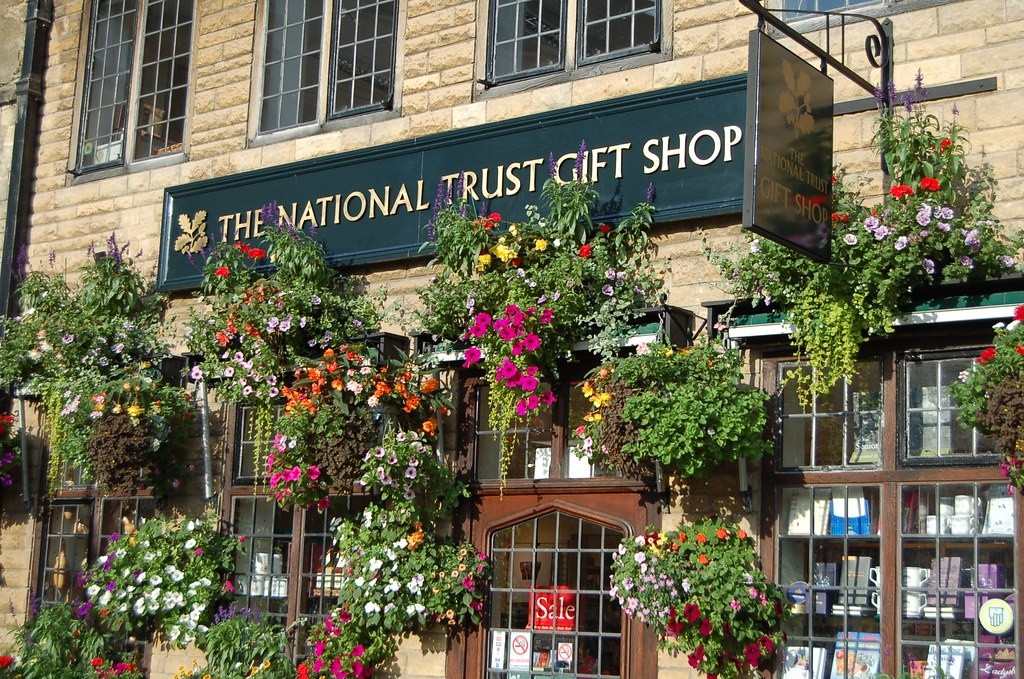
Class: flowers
0,73,1024,678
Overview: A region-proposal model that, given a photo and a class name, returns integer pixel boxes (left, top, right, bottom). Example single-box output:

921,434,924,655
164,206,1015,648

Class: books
779,631,1016,679
923,556,965,621
831,556,876,617
786,484,919,534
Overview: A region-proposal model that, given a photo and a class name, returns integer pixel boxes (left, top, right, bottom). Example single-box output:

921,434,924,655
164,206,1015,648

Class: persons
914,388,938,450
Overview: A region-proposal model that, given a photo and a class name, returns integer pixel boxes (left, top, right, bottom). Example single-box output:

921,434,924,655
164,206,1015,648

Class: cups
869,566,880,587
902,591,927,619
902,567,928,588
927,515,950,534
250,553,289,596
940,497,955,515
951,515,980,535
954,495,983,515
871,590,881,614
